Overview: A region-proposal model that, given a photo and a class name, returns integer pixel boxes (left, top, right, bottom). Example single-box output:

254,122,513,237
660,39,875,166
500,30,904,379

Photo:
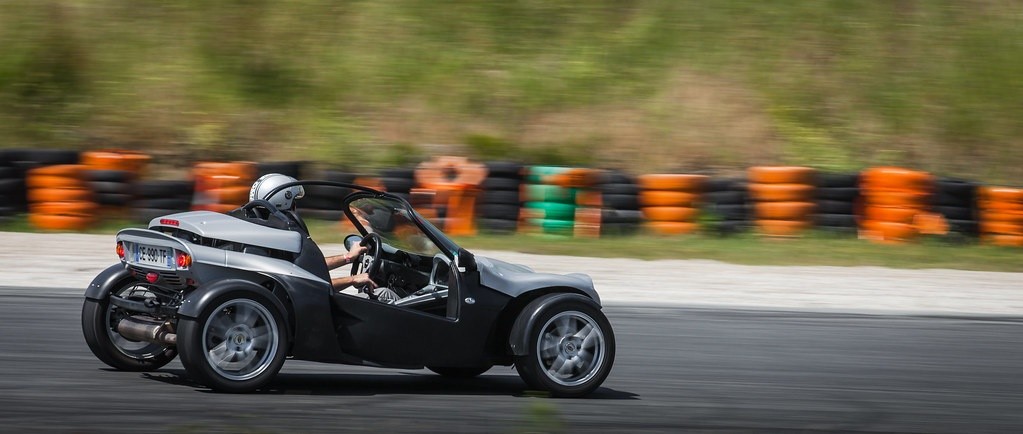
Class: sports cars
77,178,618,400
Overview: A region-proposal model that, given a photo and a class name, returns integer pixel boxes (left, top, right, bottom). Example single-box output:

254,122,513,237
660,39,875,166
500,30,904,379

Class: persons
249,173,401,304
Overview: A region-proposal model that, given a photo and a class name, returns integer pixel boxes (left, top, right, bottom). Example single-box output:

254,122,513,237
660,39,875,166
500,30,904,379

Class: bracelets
343,253,351,264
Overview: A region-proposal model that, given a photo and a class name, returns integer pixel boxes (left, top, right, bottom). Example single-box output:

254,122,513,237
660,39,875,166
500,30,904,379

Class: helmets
249,173,306,220
